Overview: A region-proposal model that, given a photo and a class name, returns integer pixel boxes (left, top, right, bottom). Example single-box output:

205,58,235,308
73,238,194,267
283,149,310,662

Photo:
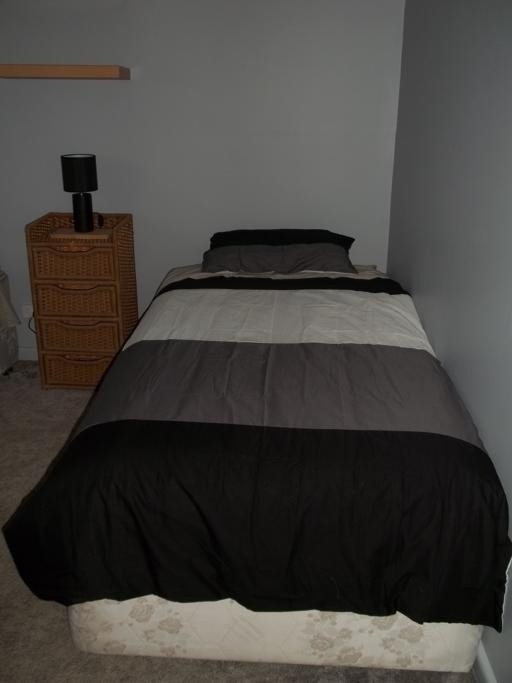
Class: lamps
58,152,100,232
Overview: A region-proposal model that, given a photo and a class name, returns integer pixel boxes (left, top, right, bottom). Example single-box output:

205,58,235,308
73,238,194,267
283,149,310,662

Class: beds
0,260,512,673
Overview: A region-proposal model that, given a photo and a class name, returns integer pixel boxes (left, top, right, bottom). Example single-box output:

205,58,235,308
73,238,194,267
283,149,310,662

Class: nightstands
23,213,139,388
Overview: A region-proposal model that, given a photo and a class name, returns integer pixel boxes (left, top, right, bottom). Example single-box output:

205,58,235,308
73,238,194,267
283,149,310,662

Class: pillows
209,228,355,253
200,245,355,273
